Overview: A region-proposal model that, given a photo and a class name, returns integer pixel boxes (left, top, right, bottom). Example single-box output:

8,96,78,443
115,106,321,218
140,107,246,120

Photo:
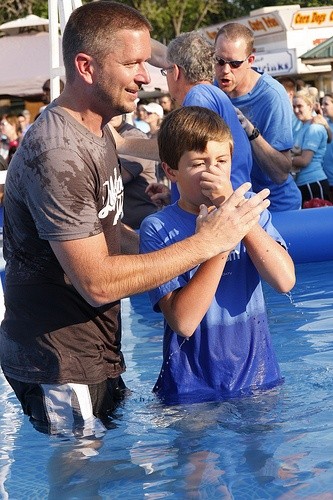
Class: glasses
215,54,249,68
160,66,174,76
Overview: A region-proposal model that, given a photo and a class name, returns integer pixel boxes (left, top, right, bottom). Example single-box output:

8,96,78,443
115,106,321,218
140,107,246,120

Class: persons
140,106,296,393
281,78,333,204
0,79,65,202
0,0,271,425
211,23,302,211
146,31,251,206
109,94,175,231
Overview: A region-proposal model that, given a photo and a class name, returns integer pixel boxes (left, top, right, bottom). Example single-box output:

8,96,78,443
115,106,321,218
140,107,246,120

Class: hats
143,103,163,118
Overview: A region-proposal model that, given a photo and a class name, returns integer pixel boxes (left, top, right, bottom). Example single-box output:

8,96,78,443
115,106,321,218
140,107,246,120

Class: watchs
248,126,260,141
